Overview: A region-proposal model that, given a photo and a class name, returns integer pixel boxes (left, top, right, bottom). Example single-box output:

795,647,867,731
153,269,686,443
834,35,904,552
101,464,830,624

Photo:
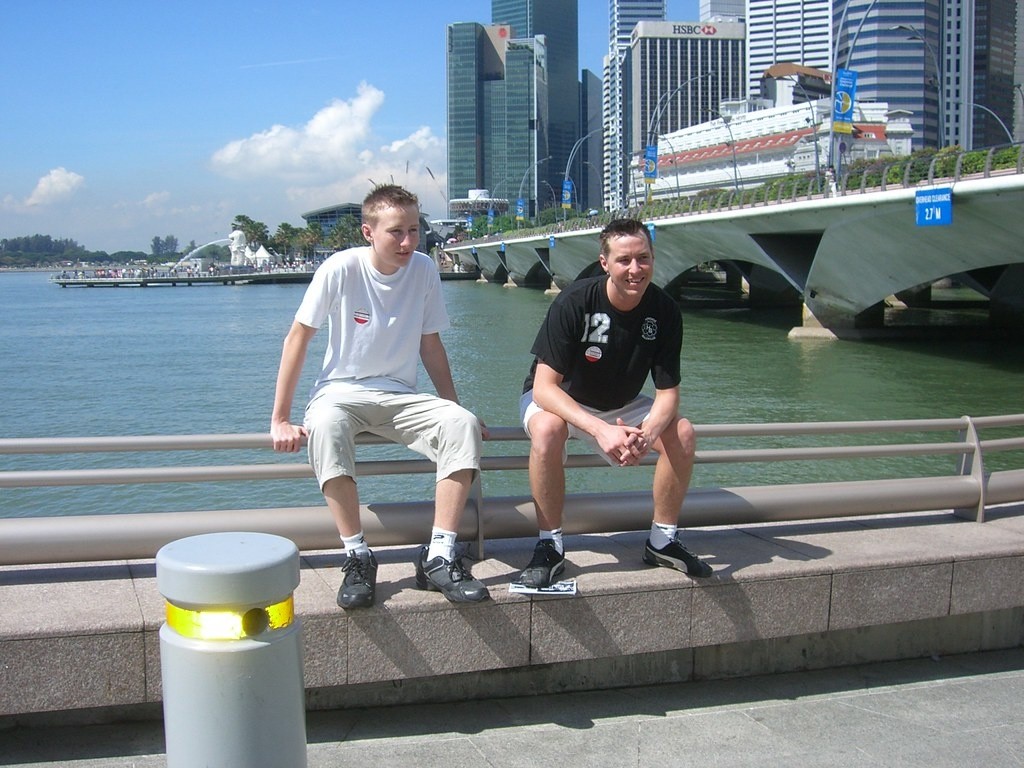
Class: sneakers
519,538,565,587
417,542,489,604
337,547,378,610
643,530,713,578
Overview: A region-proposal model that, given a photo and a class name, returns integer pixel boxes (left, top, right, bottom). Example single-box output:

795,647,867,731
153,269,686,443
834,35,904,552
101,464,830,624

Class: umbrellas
589,209,599,216
447,238,457,244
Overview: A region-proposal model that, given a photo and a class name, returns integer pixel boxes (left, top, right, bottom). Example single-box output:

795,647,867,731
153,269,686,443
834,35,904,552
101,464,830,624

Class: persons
61,259,315,279
585,212,599,229
519,220,713,588
270,183,490,609
611,206,629,224
554,219,566,234
472,230,502,240
435,241,465,273
825,166,838,199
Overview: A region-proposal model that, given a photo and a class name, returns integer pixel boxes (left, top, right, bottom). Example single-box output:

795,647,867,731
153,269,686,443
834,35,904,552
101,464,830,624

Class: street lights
646,129,681,200
561,126,605,227
468,189,488,242
487,176,513,236
952,99,1014,143
618,150,637,208
1013,83,1024,104
515,156,555,232
539,178,558,224
889,22,947,147
582,160,604,209
644,70,719,205
699,106,739,194
553,171,580,221
